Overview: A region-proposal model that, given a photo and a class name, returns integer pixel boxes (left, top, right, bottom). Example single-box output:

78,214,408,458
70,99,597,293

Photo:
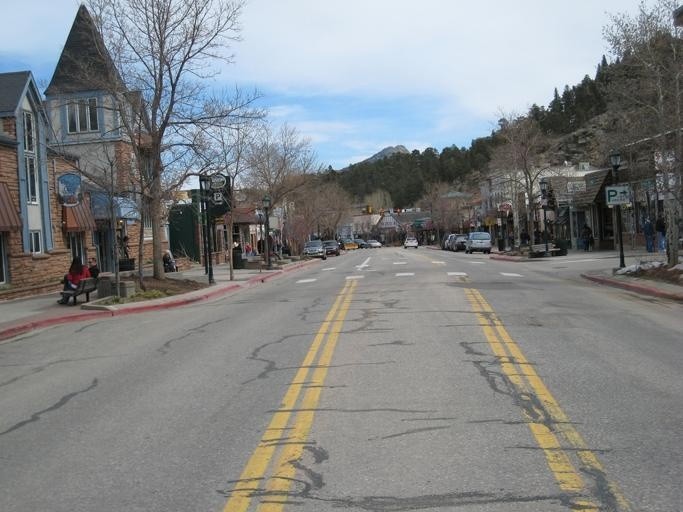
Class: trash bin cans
498,238,505,251
233,247,242,269
118,258,135,272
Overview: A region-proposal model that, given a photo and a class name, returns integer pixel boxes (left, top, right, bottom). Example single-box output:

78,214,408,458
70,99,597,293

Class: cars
354,239,382,249
302,240,340,260
444,231,492,254
404,237,418,249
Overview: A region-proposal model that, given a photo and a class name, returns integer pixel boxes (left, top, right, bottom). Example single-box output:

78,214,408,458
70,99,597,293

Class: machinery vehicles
340,239,358,250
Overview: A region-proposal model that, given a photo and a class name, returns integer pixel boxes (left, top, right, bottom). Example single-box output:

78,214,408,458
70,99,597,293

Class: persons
54,256,89,304
123,236,130,259
86,256,99,279
497,225,551,247
579,222,593,251
224,240,253,260
162,249,176,272
643,217,654,252
654,213,666,253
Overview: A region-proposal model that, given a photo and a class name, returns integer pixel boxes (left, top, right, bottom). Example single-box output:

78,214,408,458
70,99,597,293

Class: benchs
530,243,560,256
59,277,96,306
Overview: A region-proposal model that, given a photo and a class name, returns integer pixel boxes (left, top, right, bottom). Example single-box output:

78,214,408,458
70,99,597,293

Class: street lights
262,193,274,270
607,147,626,268
539,178,548,251
199,173,216,286
